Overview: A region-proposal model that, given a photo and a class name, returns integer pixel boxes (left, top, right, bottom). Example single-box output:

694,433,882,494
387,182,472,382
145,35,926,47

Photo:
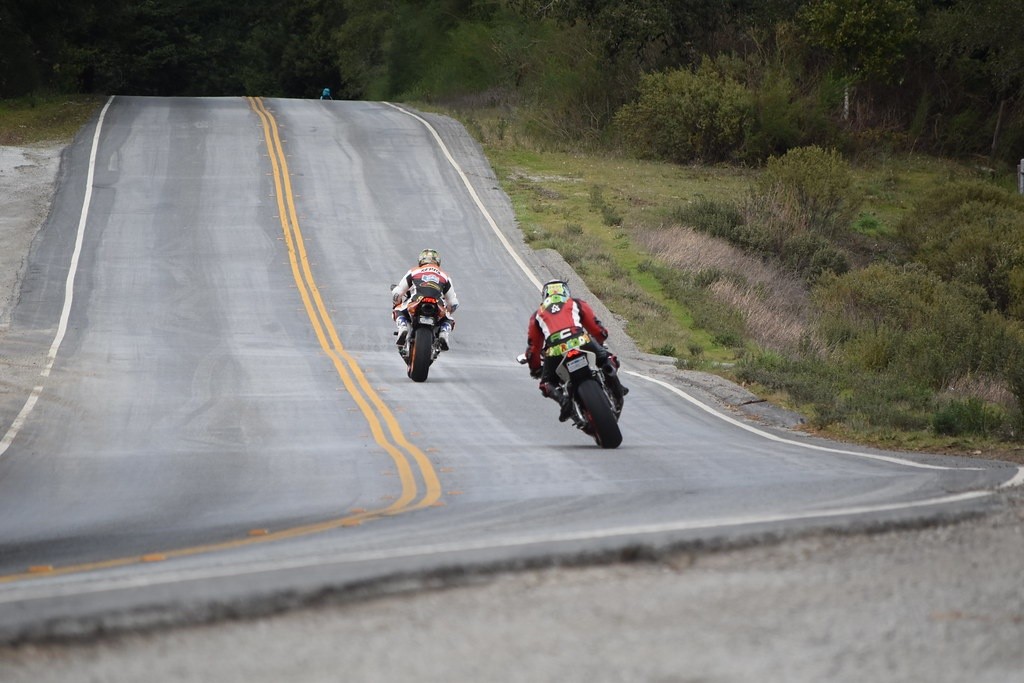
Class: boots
395,315,409,345
439,320,450,353
543,383,574,423
620,384,630,396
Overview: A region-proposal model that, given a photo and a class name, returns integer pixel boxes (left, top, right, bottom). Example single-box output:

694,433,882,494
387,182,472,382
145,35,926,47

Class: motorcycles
390,284,443,382
516,340,624,449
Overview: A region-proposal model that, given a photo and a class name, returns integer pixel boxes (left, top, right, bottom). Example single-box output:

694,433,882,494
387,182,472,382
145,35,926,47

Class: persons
392,249,460,351
525,279,629,422
320,88,333,100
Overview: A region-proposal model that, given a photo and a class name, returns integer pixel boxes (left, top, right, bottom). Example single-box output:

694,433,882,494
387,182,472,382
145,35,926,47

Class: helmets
417,247,442,265
542,278,571,296
